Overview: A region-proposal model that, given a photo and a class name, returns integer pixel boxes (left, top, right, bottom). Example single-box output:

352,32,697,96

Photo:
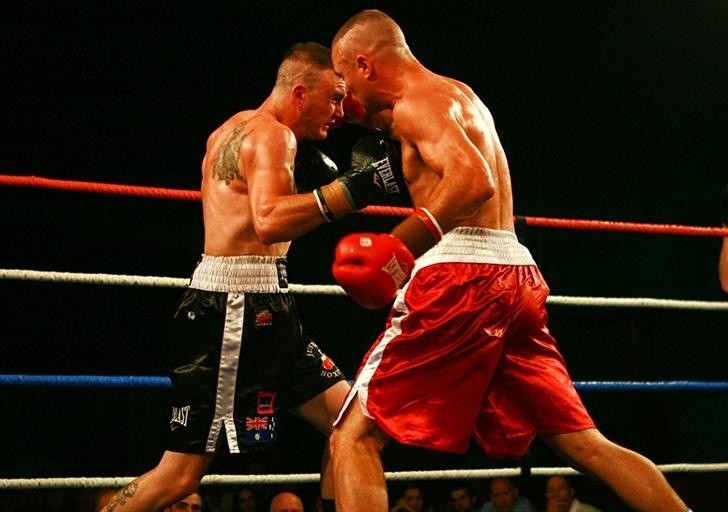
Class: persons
96,40,413,512
717,222,728,293
166,489,335,511
395,476,600,511
96,487,119,512
330,8,692,510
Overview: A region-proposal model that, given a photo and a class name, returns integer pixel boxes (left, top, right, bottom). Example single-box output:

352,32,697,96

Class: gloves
331,206,444,311
293,145,340,194
342,93,377,129
313,132,408,225
